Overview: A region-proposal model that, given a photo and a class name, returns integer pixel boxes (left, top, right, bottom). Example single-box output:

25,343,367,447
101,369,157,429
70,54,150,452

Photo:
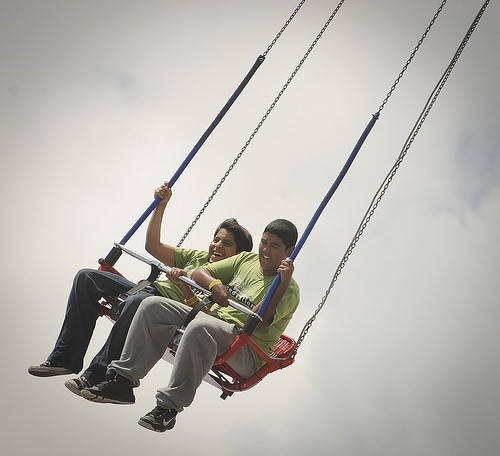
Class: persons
28,181,253,396
80,219,300,433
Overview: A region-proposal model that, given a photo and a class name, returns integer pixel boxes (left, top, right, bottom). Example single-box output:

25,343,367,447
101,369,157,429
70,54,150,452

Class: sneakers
64,375,93,395
138,405,178,432
81,369,135,404
28,360,74,376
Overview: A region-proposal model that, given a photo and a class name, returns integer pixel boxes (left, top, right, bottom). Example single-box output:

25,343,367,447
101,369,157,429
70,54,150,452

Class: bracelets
208,278,222,290
184,294,199,306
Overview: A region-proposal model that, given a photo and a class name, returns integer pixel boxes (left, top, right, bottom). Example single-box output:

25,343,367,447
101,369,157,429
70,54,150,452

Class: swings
96,0,489,400
